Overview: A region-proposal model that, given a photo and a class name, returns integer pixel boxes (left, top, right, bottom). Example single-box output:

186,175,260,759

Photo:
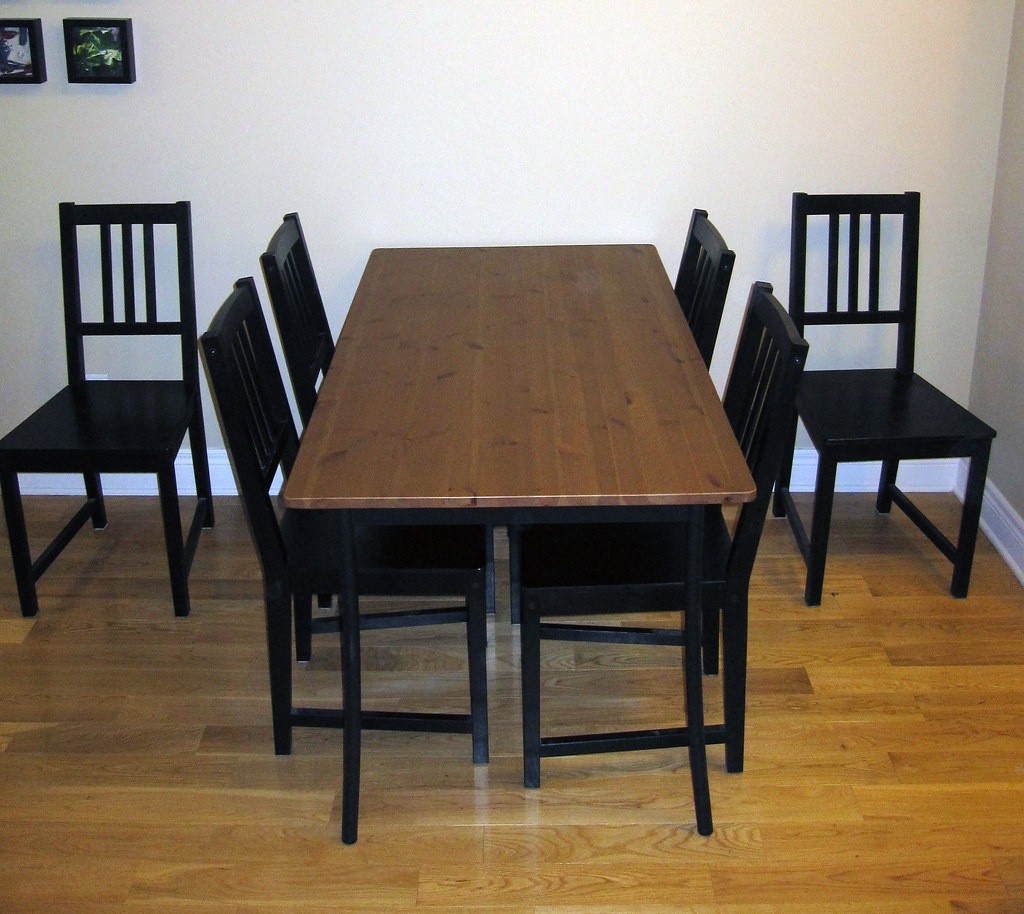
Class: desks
282,244,756,844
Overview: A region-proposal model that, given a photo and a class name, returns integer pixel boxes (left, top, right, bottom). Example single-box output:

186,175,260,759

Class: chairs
507,207,741,631
519,280,810,789
196,276,491,765
0,201,216,621
259,211,495,623
768,189,997,606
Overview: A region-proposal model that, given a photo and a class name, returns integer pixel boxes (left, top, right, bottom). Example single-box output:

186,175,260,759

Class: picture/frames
0,17,48,84
62,15,136,84
